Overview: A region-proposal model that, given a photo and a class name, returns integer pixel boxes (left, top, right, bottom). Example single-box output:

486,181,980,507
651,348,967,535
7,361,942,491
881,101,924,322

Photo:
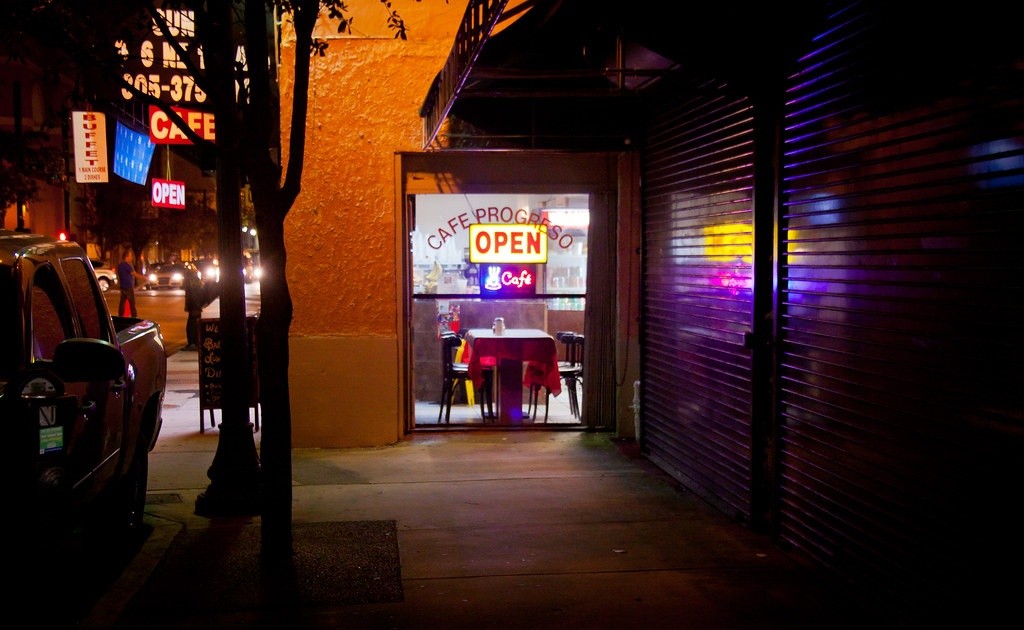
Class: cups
493,317,505,336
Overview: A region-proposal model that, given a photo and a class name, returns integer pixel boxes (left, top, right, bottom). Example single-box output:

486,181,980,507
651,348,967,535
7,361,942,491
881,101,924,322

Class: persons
183,271,202,350
118,251,149,319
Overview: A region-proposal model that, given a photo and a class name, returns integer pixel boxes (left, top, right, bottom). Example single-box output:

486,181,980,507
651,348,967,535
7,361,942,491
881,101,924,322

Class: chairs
531,335,586,424
526,329,578,416
441,330,492,419
437,335,496,424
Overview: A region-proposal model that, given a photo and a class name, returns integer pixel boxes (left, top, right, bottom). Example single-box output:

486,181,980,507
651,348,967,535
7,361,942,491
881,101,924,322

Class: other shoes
181,344,199,351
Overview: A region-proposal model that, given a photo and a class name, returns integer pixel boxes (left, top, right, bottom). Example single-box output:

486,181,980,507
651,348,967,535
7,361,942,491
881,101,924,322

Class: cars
87,246,264,299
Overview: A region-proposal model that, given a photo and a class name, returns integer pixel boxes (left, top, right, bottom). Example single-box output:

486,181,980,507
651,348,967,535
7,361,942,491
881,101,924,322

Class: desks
466,327,557,427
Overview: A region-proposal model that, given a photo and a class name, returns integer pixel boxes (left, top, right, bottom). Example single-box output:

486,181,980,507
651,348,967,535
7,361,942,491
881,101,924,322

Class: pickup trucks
1,229,168,625
